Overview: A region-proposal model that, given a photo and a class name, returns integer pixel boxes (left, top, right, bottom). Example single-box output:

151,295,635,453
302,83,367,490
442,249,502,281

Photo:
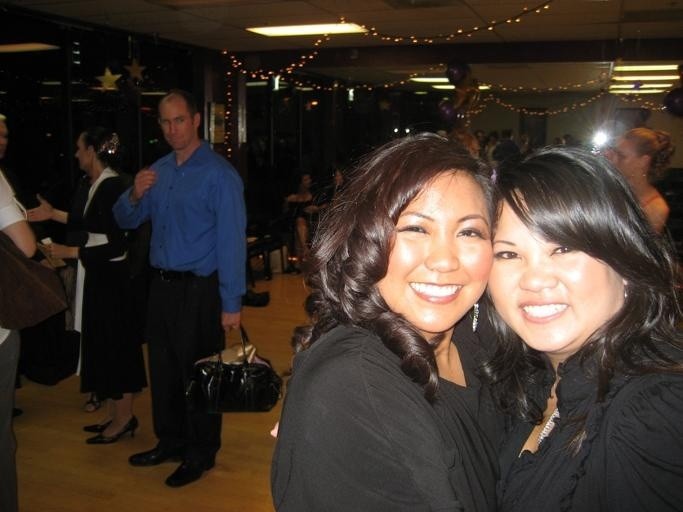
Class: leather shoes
165,458,215,488
128,440,184,466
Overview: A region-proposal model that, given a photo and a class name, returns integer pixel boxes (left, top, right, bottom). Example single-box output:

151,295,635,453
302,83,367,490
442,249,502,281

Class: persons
613,125,675,236
21,125,147,444
0,167,36,512
108,87,248,490
0,112,10,162
82,393,100,413
263,129,502,512
283,128,579,276
482,140,682,512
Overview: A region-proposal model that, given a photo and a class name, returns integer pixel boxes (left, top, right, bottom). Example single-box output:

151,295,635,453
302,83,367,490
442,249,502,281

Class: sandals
84,392,102,412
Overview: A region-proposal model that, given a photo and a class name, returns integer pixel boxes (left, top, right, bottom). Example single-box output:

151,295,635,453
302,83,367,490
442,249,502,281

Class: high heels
83,416,139,443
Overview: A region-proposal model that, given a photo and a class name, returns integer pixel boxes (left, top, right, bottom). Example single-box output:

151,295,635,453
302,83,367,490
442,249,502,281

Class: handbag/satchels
184,356,283,413
